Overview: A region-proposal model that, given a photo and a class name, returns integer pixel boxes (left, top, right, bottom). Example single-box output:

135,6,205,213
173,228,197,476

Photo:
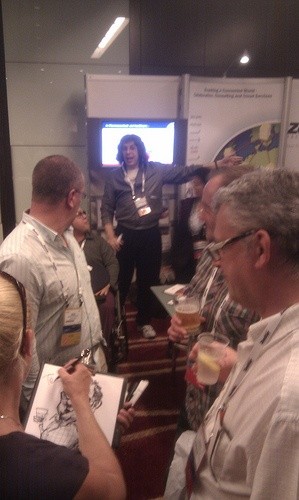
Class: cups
195,333,230,386
172,292,202,335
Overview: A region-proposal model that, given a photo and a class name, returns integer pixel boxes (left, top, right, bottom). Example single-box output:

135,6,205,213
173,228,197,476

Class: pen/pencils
53,348,90,382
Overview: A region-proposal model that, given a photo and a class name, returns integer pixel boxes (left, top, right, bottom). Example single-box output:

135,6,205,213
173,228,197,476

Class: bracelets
214,160,217,170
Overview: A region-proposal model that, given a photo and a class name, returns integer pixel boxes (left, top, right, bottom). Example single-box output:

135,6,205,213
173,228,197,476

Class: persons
100,137,244,345
0,154,299,500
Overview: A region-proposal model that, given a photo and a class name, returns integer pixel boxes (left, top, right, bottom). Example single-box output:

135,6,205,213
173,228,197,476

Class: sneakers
138,324,156,338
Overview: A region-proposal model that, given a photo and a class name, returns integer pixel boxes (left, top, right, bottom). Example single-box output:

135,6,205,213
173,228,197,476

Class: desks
150,285,201,374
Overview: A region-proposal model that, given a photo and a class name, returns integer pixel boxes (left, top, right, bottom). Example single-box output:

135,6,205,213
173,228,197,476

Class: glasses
0,270,26,352
66,187,86,199
208,229,276,261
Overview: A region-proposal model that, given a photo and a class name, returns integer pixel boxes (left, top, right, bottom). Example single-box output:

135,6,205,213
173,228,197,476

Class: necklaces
0,415,21,427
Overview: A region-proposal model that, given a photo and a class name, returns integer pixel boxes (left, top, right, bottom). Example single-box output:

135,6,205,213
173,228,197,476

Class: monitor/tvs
87,118,188,171
